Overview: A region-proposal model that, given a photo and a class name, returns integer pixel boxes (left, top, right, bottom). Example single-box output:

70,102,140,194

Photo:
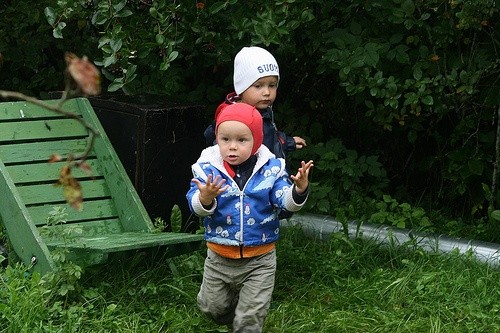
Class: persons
185,101,315,333
202,46,307,159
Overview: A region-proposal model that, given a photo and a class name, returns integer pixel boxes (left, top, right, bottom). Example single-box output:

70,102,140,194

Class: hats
214,102,264,158
233,46,280,95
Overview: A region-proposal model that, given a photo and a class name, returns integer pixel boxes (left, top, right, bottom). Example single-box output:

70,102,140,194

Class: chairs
0,98,207,274
